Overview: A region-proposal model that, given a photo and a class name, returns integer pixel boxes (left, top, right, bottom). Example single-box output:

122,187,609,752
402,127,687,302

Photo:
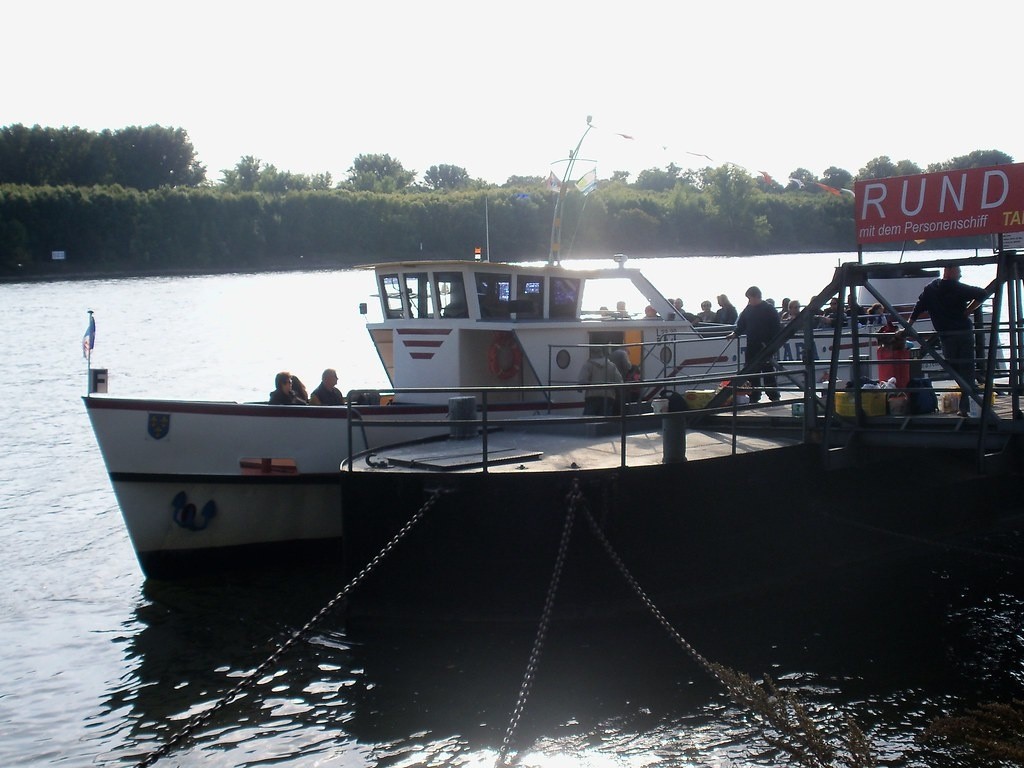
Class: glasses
285,379,293,385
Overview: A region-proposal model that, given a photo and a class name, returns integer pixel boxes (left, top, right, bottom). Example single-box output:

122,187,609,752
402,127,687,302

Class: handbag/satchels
887,391,907,416
659,390,687,412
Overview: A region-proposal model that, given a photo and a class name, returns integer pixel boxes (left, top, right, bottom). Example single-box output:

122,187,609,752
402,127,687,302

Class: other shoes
749,396,760,403
957,411,967,416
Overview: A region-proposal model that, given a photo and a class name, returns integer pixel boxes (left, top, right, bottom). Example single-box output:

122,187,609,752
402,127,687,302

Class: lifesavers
487,331,525,383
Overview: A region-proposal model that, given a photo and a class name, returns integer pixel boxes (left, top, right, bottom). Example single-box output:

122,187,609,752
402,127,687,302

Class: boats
81,125,1012,617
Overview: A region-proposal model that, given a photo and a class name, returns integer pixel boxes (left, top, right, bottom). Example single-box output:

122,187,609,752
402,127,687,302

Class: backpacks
908,376,938,414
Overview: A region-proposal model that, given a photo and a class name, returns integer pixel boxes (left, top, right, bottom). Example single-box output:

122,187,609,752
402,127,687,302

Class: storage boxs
685,390,715,409
836,390,887,417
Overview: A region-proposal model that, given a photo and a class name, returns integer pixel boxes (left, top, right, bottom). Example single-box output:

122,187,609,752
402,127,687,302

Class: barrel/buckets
652,399,669,414
968,395,984,418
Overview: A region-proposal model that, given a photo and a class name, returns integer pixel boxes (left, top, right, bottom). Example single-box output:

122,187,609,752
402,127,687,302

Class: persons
597,295,888,328
578,347,642,417
895,266,990,417
269,369,344,406
726,286,781,403
